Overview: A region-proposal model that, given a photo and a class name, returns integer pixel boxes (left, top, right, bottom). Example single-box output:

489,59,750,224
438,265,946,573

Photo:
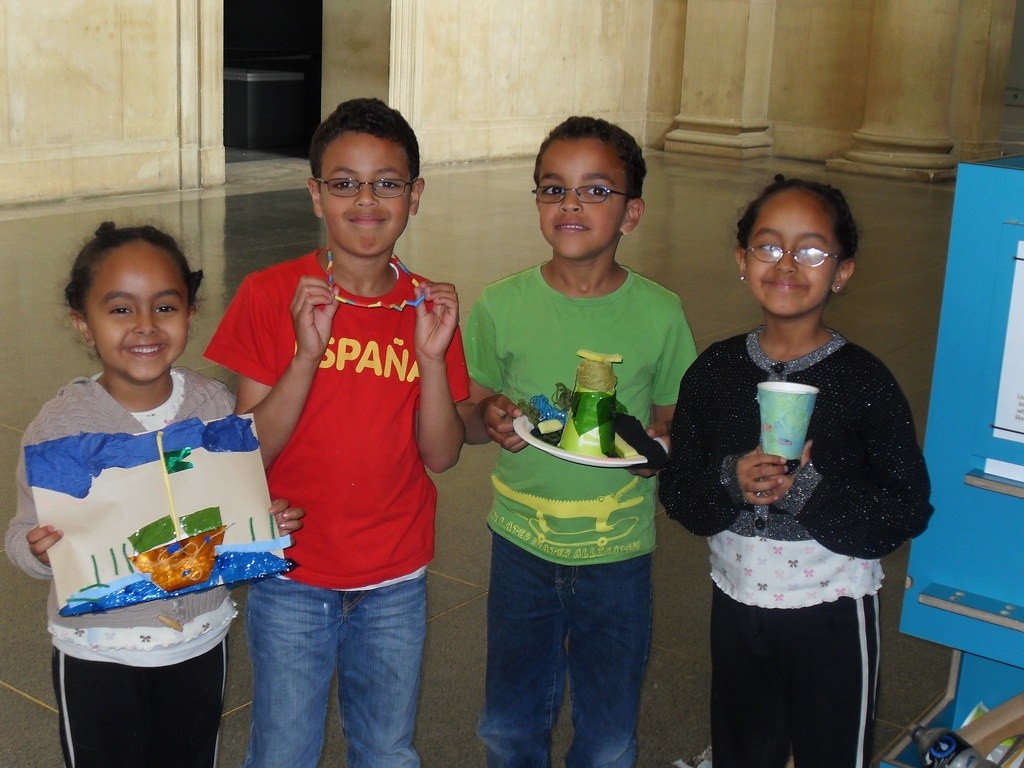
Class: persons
457,116,698,768
203,99,469,768
660,177,934,768
5,222,304,768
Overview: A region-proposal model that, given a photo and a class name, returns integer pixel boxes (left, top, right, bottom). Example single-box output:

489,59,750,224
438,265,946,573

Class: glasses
532,185,631,204
314,178,413,198
743,243,845,267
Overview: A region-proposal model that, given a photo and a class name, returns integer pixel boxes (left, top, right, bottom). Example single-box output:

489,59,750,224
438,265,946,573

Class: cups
757,381,820,461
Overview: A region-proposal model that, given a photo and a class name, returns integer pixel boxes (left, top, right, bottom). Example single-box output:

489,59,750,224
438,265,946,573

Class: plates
513,414,669,468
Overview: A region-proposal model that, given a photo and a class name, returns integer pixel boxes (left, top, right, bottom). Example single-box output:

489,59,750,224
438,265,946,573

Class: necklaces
325,245,426,312
